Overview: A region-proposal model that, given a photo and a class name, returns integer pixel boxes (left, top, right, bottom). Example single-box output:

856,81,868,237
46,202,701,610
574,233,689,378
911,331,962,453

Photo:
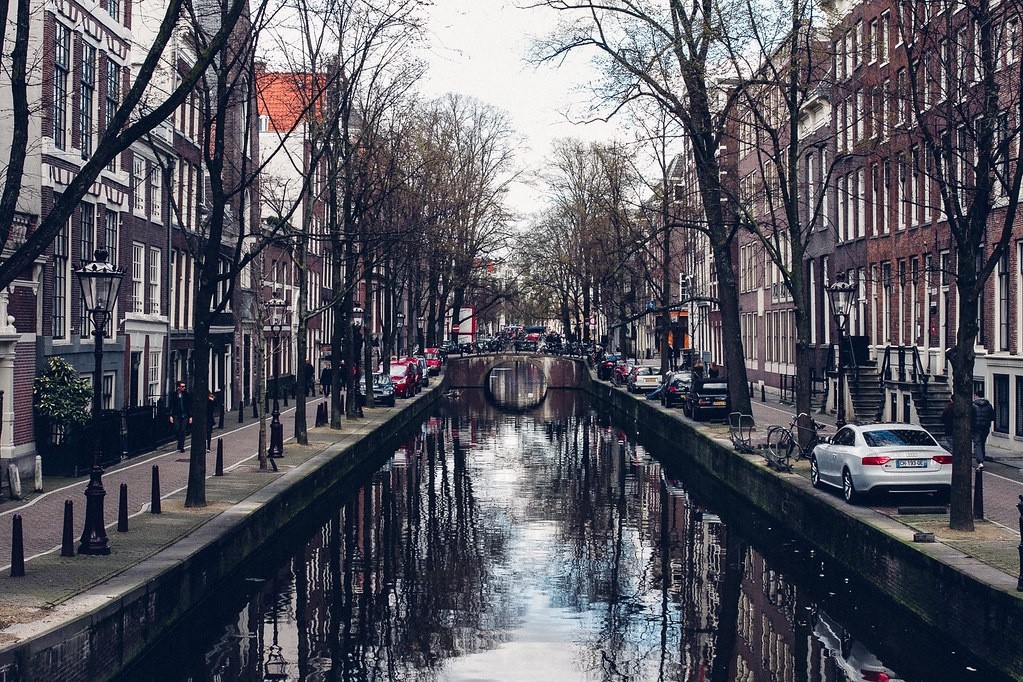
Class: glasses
182,386,186,388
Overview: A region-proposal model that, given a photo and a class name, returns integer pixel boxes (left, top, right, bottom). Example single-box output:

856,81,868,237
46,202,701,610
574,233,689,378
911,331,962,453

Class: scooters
460,324,604,373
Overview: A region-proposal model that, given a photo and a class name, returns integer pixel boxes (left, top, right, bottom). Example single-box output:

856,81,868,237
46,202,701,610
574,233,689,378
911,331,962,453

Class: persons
320,364,331,398
972,390,996,468
941,394,954,452
695,359,704,379
709,362,720,378
168,381,192,452
306,358,314,396
206,390,215,453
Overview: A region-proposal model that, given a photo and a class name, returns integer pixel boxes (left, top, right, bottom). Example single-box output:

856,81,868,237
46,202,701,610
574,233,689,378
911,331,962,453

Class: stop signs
452,324,460,333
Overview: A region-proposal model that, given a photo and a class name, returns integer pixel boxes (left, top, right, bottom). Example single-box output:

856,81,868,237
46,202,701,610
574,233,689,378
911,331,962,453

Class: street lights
353,302,365,419
394,311,405,360
416,314,426,355
824,271,856,431
264,290,288,459
74,242,128,556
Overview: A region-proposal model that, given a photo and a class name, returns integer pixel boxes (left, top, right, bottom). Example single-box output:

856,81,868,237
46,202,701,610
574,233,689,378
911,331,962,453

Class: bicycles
767,412,833,460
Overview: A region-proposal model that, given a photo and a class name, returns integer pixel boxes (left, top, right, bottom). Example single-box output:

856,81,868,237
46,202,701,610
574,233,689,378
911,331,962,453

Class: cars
356,372,398,407
597,352,627,381
682,379,733,421
810,422,953,505
609,360,641,386
626,364,665,394
658,371,693,409
392,340,456,393
374,362,416,399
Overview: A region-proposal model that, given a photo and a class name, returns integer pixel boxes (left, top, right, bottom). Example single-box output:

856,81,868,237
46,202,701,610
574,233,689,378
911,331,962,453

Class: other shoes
180,447,185,453
976,463,984,471
206,449,210,453
